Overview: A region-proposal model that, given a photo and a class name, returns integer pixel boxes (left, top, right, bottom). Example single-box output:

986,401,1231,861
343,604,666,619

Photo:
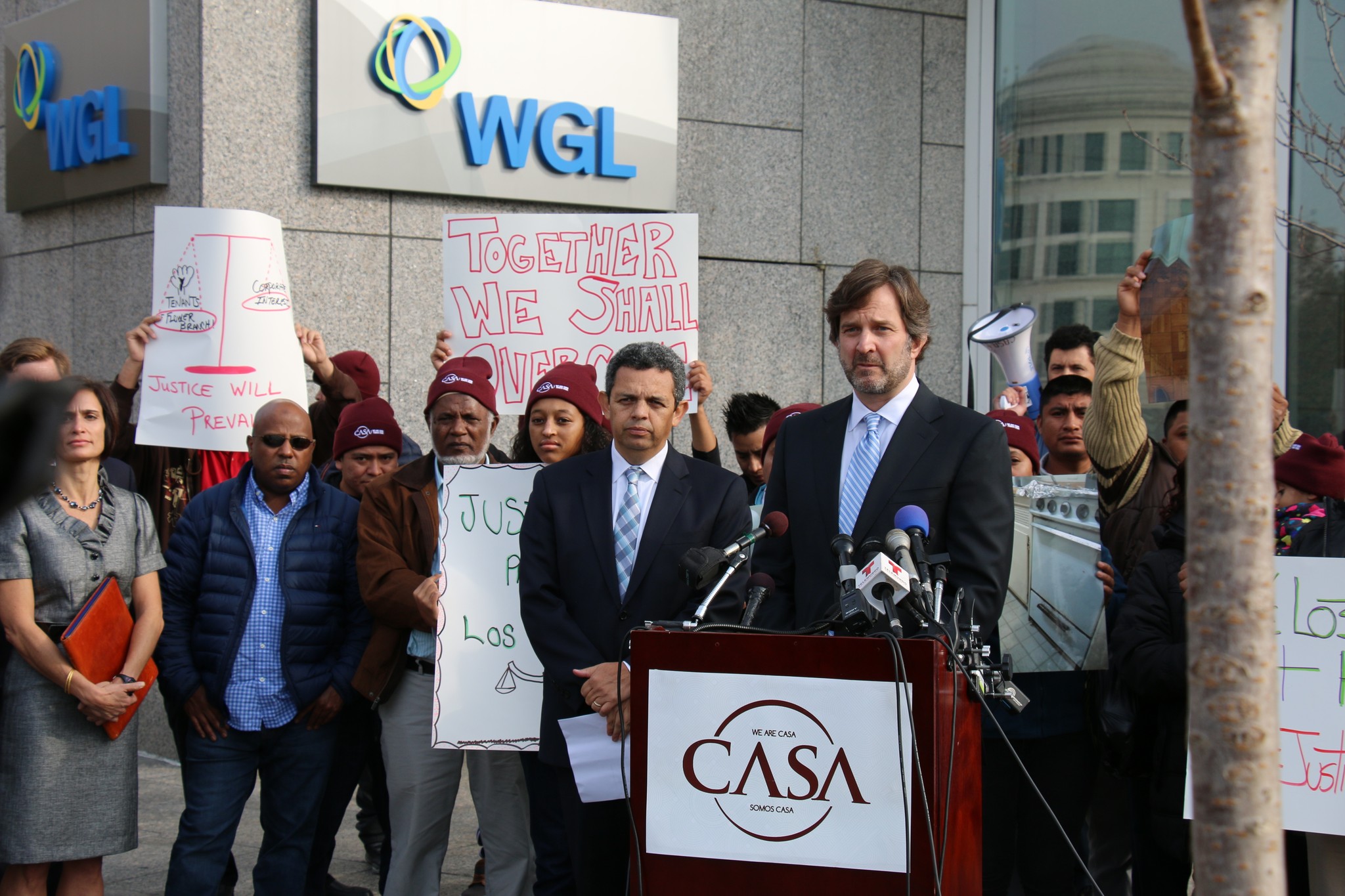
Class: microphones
740,572,776,630
724,510,788,556
830,505,966,635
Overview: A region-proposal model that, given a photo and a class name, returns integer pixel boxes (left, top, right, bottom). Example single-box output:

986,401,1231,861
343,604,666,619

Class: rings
593,700,602,707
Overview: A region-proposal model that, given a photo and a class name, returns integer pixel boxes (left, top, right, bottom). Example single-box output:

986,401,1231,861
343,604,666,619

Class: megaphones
968,305,1044,422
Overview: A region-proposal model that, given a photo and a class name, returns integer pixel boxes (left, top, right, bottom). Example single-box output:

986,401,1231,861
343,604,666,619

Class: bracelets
64,667,74,696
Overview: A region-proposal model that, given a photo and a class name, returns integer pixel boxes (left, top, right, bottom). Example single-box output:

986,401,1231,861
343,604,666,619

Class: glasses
251,433,315,450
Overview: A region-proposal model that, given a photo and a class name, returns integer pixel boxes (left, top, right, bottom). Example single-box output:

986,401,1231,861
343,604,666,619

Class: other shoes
363,843,384,873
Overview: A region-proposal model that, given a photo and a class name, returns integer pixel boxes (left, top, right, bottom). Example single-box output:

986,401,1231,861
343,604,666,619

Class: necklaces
49,475,104,511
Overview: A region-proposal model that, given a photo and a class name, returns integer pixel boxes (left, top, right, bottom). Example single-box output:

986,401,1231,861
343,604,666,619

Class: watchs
111,673,138,698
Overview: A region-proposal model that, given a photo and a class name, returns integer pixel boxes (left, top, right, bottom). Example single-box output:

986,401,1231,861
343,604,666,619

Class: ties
838,413,884,539
614,466,644,605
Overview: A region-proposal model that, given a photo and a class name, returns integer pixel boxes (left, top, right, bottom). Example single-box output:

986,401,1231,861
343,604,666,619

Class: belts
402,656,435,675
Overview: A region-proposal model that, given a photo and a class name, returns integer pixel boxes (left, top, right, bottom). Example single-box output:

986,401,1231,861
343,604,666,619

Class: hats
760,403,821,468
424,357,500,416
524,361,602,427
312,351,381,401
1276,432,1345,503
332,396,402,460
986,410,1041,475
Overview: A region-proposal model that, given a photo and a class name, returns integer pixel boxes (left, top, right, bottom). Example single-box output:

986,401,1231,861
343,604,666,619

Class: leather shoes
308,881,373,896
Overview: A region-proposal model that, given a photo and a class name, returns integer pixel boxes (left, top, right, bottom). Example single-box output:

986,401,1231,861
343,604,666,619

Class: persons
1273,428,1345,558
0,379,172,896
462,355,608,896
514,337,757,896
105,315,363,896
745,399,826,521
307,398,424,895
751,257,1018,687
1106,451,1191,896
301,345,425,478
432,324,727,469
718,388,777,506
140,394,366,896
0,335,71,398
981,407,1043,482
1041,319,1105,388
352,348,543,896
1079,245,1310,896
991,374,1098,480
